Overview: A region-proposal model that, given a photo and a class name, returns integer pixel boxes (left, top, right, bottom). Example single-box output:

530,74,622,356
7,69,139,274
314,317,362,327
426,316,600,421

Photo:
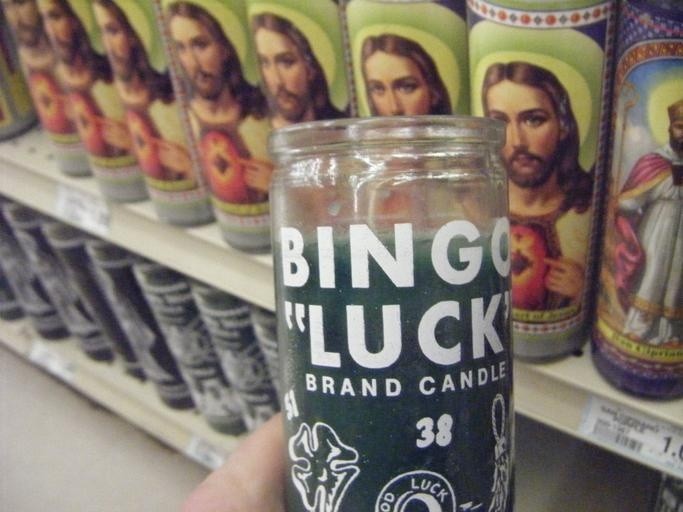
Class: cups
259,114,516,511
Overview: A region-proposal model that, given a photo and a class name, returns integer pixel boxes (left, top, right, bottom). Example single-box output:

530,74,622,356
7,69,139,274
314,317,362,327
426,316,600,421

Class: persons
178,409,290,511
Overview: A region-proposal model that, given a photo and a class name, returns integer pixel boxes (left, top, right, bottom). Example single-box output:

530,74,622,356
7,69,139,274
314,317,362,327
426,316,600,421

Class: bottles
1,2,683,451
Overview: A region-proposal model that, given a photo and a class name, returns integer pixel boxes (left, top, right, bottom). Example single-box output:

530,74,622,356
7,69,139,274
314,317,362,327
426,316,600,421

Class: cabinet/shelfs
0,117,682,512
